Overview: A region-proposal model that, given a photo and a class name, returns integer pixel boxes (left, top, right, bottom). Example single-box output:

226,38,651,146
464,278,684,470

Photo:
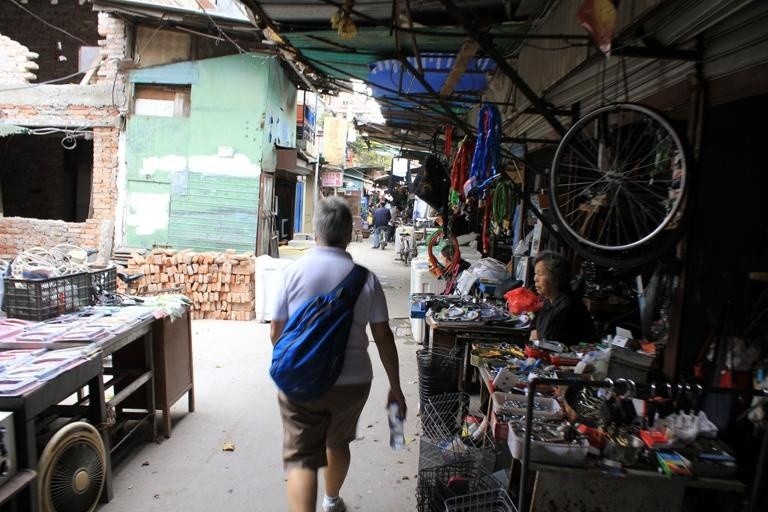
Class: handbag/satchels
269,263,370,404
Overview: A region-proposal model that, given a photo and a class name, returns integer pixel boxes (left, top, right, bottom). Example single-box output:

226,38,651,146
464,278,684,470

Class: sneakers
322,495,344,511
372,242,385,249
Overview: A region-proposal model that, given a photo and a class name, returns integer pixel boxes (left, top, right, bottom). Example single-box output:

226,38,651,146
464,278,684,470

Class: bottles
438,416,488,464
387,396,406,449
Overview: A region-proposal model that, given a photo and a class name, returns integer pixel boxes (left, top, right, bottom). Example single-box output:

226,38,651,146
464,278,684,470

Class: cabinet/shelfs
0,296,194,511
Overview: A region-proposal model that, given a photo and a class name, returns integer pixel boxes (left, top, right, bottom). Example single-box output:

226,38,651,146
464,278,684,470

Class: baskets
416,347,519,512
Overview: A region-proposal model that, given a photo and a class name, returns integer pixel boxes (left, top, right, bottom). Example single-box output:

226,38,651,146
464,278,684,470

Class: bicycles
399,232,412,264
379,227,387,250
353,228,361,241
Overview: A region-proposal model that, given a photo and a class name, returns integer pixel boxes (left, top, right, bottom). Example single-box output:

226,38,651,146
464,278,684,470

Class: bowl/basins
603,433,644,466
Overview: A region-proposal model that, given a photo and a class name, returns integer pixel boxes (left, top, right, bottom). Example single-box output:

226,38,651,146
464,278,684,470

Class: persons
440,245,472,286
526,249,596,346
366,197,403,249
268,196,409,512
